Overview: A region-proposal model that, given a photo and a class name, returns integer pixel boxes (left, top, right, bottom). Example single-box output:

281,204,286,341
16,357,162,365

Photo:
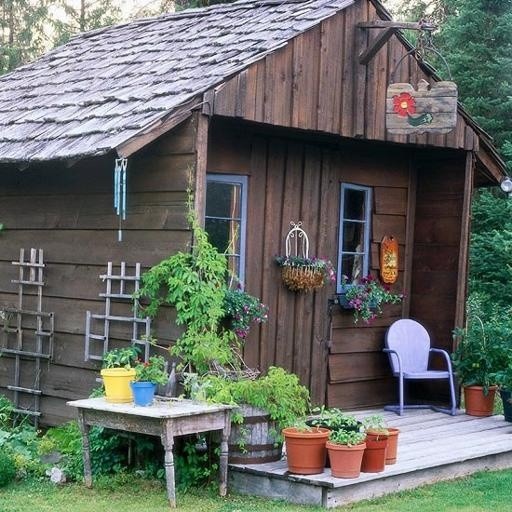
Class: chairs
384,317,458,416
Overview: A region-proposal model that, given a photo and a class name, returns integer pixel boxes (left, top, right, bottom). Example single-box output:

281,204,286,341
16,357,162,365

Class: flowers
278,251,406,323
391,94,433,128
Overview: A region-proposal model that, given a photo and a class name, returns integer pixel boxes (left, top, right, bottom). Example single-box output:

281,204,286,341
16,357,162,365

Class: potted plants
195,370,399,478
451,292,512,421
98,350,168,405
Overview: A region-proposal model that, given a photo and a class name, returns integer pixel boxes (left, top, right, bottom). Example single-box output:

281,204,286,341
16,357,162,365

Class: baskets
281,228,324,296
